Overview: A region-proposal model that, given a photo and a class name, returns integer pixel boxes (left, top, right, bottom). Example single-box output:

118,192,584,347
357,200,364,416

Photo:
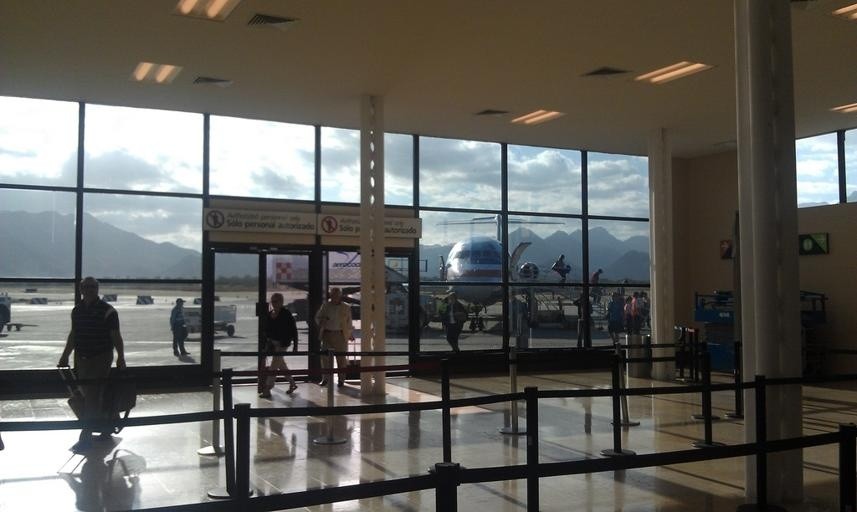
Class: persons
253,416,298,496
444,292,468,353
313,288,355,387
259,293,298,398
57,457,142,512
551,254,649,347
170,298,191,356
59,277,127,455
508,290,530,348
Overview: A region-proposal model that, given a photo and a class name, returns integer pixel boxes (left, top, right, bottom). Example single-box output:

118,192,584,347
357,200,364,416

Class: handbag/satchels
105,447,147,480
106,368,138,413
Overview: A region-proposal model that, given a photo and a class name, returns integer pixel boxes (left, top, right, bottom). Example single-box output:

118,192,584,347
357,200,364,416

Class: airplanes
420,213,567,331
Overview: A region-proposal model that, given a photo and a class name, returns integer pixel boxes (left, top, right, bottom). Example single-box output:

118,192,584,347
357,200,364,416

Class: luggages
54,435,124,476
56,361,122,433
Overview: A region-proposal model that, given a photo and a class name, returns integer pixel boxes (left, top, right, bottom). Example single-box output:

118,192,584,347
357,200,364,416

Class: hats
175,298,187,302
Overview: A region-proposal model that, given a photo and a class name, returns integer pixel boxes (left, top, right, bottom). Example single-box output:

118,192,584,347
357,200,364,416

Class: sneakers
257,384,298,398
317,379,345,388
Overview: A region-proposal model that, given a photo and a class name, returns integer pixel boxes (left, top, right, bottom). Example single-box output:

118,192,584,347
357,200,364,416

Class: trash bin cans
626,334,650,378
